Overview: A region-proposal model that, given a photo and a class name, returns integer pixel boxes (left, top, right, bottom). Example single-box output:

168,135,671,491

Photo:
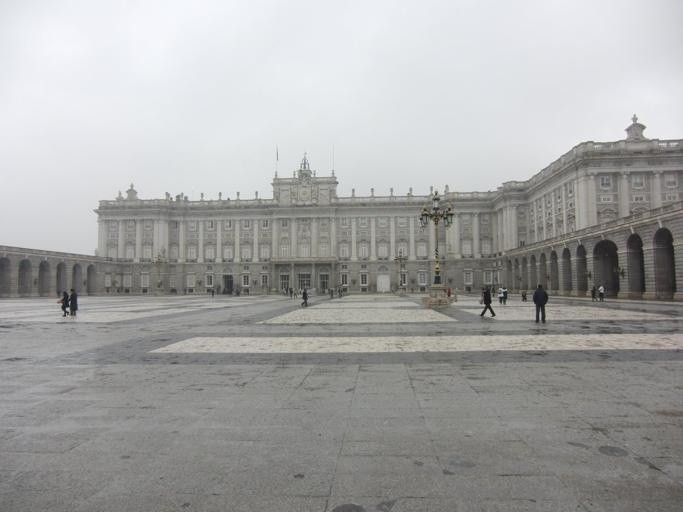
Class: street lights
417,189,456,310
393,247,408,297
154,255,164,296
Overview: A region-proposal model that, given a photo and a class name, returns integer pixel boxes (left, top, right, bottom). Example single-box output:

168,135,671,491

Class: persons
68,289,77,317
502,287,508,305
532,284,549,323
591,287,599,302
498,286,508,304
60,291,69,317
300,288,309,308
479,286,496,317
521,290,526,301
598,284,605,303
